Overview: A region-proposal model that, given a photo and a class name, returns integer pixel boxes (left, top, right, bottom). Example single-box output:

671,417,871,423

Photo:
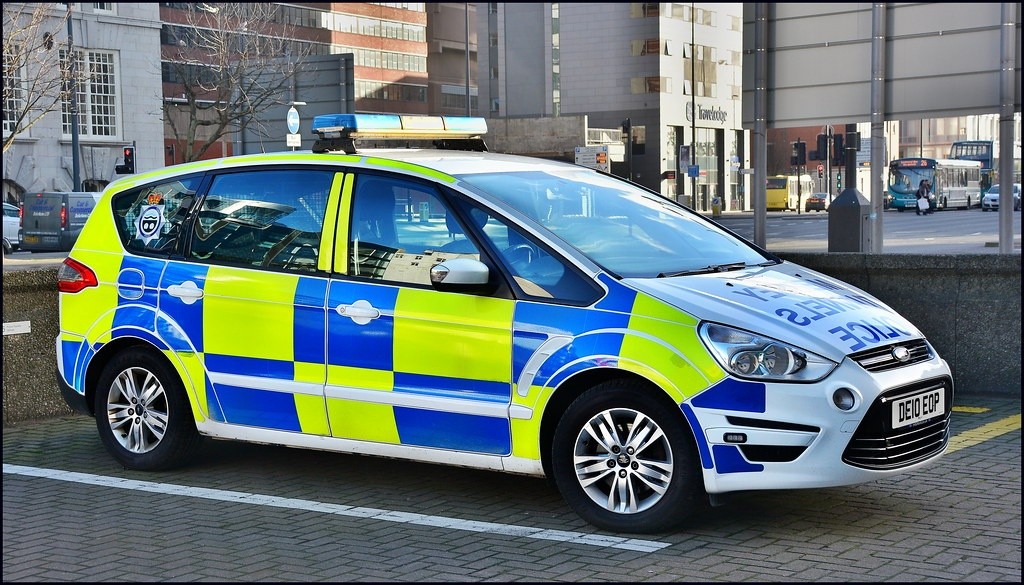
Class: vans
18,191,103,254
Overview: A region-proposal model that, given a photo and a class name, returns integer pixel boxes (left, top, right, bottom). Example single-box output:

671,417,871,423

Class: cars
805,193,836,213
981,183,1022,212
2,203,22,252
53,110,954,534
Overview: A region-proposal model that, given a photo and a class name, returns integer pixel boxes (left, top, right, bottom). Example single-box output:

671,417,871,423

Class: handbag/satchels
917,195,929,210
915,186,925,199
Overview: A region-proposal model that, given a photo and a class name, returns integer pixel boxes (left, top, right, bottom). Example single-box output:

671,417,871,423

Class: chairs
441,205,488,254
356,181,400,251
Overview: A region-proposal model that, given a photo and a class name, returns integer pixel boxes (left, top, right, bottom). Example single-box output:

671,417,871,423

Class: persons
925,179,934,214
915,180,929,215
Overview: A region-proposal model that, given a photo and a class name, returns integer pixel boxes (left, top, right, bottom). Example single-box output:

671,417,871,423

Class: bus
886,157,982,213
765,174,813,211
947,140,1021,201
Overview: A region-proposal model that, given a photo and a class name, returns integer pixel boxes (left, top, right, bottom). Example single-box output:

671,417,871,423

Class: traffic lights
817,165,825,179
115,164,126,175
123,146,136,174
836,182,841,189
837,173,841,181
